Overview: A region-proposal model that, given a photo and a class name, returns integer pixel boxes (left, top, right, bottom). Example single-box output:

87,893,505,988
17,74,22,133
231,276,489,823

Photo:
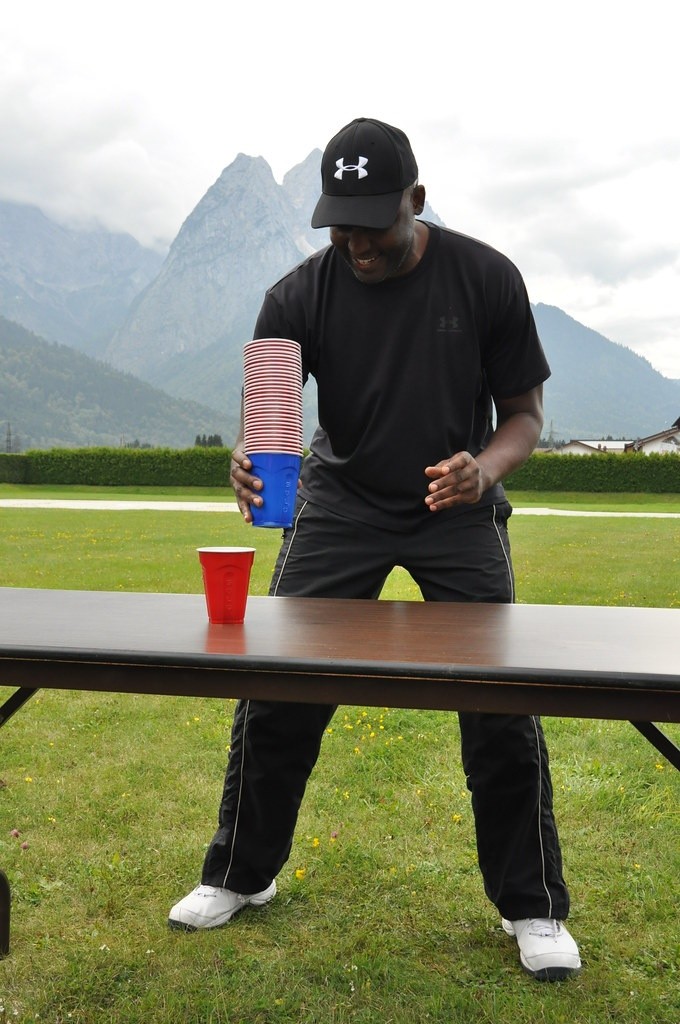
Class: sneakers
502,915,581,978
168,878,277,930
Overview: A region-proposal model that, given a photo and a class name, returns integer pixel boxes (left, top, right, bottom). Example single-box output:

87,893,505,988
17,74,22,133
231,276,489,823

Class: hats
311,117,419,230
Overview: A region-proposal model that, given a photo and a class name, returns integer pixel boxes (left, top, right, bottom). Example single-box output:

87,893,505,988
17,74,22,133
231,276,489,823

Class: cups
242,339,302,528
197,547,256,623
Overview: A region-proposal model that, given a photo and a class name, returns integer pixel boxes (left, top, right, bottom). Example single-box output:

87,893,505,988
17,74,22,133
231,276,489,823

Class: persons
167,117,582,978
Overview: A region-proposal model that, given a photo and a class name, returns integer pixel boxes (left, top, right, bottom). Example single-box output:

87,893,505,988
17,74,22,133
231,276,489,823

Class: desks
0,585,680,958
